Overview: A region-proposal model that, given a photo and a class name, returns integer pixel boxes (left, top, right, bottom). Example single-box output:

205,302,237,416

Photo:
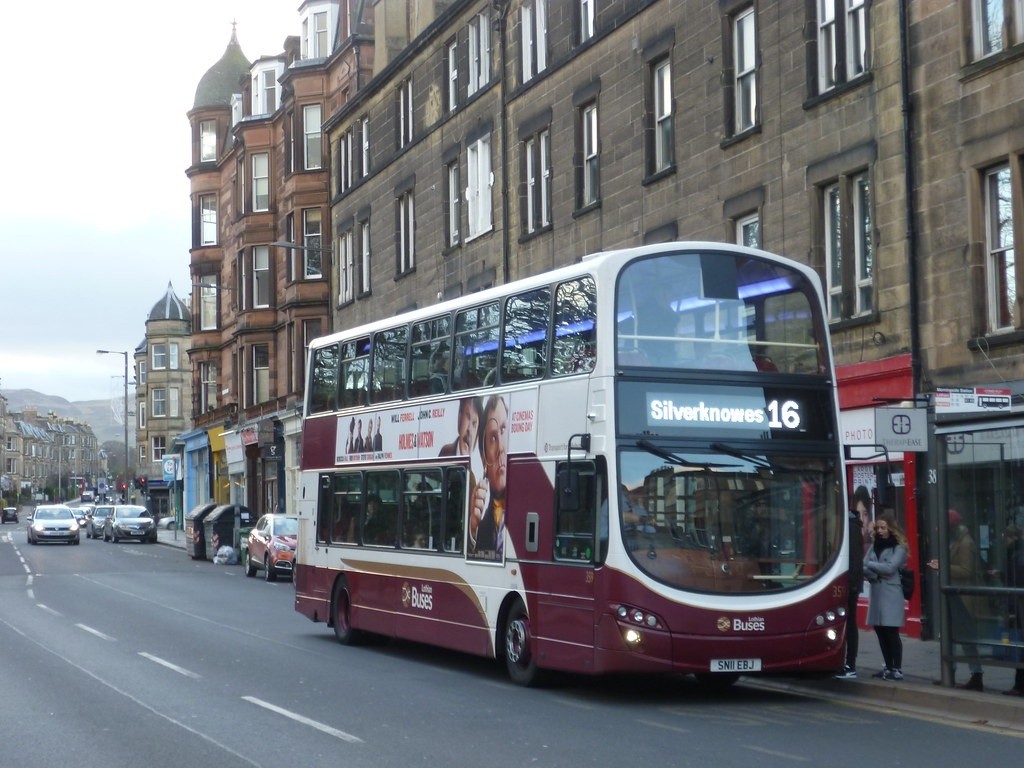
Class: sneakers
835,665,856,679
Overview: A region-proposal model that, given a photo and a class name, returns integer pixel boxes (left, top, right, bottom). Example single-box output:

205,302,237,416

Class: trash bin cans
238,527,253,564
184,503,218,558
203,504,248,561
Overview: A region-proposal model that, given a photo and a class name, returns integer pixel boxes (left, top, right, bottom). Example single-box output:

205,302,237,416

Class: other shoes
1001,685,1024,697
957,672,983,692
881,667,904,679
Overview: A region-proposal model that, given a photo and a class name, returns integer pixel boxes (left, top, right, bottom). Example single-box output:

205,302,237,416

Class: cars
79,505,95,519
26,505,80,545
69,508,88,527
245,513,299,582
84,506,114,539
2,508,19,523
103,505,157,544
79,491,93,502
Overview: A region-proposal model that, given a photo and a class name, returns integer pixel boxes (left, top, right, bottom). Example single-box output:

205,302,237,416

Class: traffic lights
140,477,145,484
121,484,126,489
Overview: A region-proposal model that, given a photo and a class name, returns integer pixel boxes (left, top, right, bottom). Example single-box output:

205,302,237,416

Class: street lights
97,349,129,483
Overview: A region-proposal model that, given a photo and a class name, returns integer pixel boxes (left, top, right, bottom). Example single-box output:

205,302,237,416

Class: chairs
743,354,778,373
358,384,381,406
415,376,430,396
345,389,360,407
702,352,738,370
618,347,650,366
327,391,345,410
377,382,395,403
393,379,416,399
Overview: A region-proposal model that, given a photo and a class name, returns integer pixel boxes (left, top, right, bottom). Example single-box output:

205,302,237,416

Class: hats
948,510,962,526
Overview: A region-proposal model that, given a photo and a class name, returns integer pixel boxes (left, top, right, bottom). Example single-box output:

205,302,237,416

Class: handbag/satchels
893,543,915,600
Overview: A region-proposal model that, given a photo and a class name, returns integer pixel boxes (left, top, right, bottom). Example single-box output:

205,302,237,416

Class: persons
428,358,448,393
835,509,863,679
410,482,458,548
927,510,983,691
988,535,1024,695
345,416,382,454
854,485,873,544
863,513,911,680
363,493,387,544
438,396,508,565
600,483,648,562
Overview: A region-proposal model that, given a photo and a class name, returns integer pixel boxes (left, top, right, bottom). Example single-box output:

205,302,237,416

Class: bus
294,240,894,687
